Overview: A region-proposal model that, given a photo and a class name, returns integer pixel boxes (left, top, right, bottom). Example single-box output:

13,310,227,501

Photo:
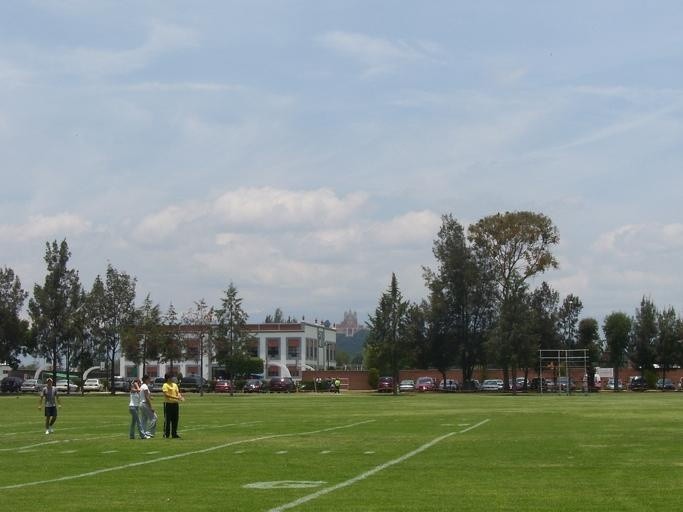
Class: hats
47,378,53,382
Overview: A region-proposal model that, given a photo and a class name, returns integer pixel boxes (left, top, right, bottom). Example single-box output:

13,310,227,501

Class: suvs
378,377,394,393
582,374,601,391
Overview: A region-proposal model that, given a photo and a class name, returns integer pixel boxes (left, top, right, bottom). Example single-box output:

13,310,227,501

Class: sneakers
45,429,50,435
146,431,154,437
172,435,180,438
163,433,169,438
51,428,53,433
143,435,150,439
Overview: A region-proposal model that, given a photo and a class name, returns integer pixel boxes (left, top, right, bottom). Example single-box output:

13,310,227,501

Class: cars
677,377,683,391
396,379,416,392
439,379,457,392
462,379,504,393
607,378,623,390
0,374,297,394
415,377,436,392
509,376,566,390
626,376,649,390
655,378,676,391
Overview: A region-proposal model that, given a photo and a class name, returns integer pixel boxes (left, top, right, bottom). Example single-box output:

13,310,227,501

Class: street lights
567,301,573,394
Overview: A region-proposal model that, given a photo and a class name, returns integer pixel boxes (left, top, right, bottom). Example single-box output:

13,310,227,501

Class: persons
161,372,185,438
37,378,60,434
313,375,342,393
140,375,159,437
128,378,149,439
